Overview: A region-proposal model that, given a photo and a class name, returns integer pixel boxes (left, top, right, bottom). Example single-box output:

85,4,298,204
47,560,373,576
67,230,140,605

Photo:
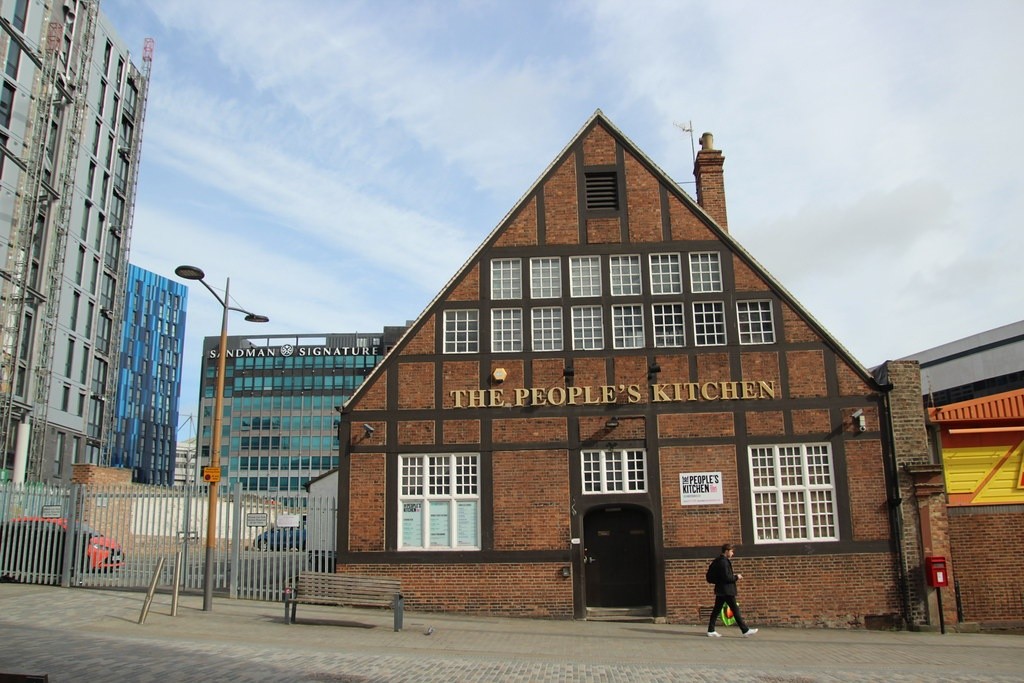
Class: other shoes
742,628,758,637
707,631,722,638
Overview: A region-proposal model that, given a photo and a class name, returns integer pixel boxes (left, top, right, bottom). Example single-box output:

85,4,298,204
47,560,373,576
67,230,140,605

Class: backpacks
705,558,726,584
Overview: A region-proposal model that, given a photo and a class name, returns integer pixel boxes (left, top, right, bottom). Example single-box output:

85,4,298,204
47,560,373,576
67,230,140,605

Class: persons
707,543,759,637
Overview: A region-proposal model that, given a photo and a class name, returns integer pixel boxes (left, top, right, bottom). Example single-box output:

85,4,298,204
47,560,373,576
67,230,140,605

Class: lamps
562,363,662,430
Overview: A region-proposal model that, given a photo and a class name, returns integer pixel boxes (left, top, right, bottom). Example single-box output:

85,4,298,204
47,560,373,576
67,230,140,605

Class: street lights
176,265,270,611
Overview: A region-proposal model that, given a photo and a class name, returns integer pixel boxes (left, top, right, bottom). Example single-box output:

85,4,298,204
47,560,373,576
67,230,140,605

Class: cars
0,517,126,572
252,526,306,552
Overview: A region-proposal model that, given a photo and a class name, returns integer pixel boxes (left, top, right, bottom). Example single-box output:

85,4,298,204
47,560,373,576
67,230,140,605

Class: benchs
284,571,405,631
